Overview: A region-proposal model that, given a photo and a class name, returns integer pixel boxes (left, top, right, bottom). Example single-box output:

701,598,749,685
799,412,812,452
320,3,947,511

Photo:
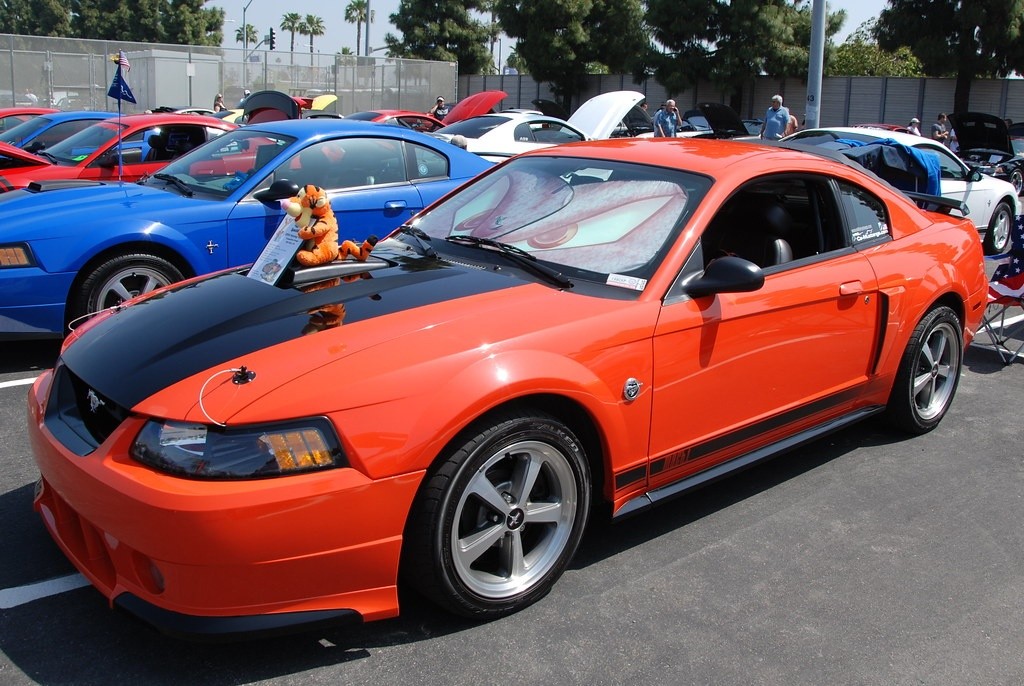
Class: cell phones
673,108,677,112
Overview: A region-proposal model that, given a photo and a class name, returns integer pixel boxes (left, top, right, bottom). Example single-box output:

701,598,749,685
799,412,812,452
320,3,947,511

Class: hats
244,89,250,94
911,118,920,123
437,97,445,102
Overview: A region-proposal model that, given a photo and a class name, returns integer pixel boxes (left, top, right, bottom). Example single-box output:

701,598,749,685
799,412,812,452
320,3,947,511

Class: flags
110,49,130,72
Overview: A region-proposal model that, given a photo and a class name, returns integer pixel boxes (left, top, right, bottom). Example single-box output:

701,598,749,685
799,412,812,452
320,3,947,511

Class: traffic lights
270,28,276,50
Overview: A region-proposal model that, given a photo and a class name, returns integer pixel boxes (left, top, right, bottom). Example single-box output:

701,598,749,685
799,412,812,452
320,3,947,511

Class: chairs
297,148,334,189
976,214,1024,365
726,185,792,267
336,153,376,187
143,137,162,161
170,142,190,158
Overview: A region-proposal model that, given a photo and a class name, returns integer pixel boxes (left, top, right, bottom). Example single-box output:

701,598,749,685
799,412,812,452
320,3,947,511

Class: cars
0,119,497,351
777,126,1019,257
149,93,337,137
850,113,1024,195
346,89,508,135
429,90,646,163
504,98,765,140
0,107,126,158
0,115,346,204
25,135,989,641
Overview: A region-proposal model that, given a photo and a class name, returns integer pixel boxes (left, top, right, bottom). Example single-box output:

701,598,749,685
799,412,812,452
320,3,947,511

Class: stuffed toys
282,184,377,265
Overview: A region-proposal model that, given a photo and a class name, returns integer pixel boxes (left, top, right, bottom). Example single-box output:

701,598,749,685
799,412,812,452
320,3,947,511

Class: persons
949,128,960,153
931,112,949,145
761,95,789,143
239,89,251,104
213,93,227,112
641,103,648,110
1004,118,1013,129
653,100,683,138
907,118,921,137
431,96,451,120
24,87,38,102
785,114,798,137
655,100,682,137
